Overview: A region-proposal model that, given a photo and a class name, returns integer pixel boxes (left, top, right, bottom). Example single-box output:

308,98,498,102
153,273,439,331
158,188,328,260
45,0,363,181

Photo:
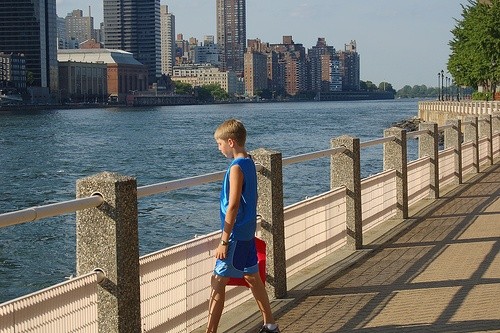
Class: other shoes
257,323,280,332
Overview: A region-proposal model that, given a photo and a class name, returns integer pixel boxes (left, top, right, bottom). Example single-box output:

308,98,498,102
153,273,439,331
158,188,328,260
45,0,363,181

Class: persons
205,119,281,333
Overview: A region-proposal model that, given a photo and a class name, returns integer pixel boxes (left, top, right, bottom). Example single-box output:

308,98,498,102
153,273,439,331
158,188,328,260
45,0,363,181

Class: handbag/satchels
226,237,266,288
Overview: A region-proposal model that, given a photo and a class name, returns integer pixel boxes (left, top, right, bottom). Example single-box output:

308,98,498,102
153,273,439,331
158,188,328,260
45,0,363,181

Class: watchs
220,240,228,246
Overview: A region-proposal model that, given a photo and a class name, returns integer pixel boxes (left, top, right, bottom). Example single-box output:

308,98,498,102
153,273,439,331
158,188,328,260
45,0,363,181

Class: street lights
438,73,440,101
441,70,444,100
446,76,448,100
